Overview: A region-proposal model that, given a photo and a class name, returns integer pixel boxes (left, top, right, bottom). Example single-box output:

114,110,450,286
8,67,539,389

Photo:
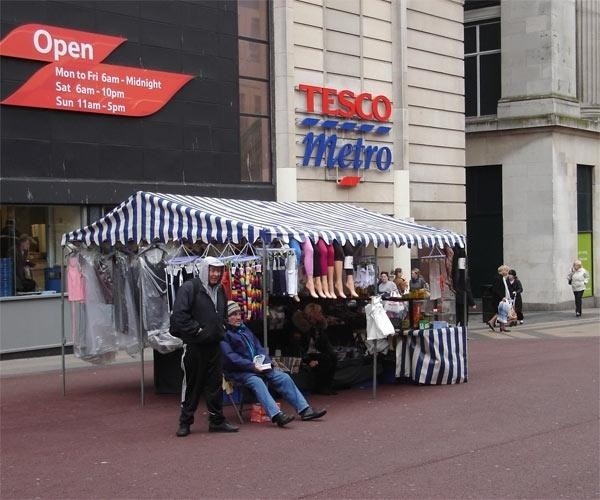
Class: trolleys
507,291,518,328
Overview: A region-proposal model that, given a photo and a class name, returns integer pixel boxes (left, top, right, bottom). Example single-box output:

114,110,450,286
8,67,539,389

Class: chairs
221,376,282,423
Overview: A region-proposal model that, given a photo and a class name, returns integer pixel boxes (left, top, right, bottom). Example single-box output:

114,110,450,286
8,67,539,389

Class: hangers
63,242,202,262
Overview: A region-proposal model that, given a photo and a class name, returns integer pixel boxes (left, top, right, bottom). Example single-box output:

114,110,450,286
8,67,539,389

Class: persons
567,260,589,316
286,302,339,396
277,239,360,302
409,268,427,291
487,264,514,332
393,268,408,295
506,269,524,325
1,220,21,258
10,233,37,291
220,300,327,426
378,272,398,295
456,279,476,339
168,255,240,437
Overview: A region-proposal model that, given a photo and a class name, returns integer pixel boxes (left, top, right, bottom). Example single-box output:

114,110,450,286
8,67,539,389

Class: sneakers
300,409,327,421
175,426,190,437
208,420,239,433
276,413,294,426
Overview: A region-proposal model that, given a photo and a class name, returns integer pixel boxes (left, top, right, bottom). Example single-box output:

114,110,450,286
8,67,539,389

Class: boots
500,323,511,332
488,316,496,332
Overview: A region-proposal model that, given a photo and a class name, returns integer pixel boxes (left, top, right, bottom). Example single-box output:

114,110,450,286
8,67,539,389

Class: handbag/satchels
169,314,181,337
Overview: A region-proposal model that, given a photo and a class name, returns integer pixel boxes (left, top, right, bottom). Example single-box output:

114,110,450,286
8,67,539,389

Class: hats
227,300,240,317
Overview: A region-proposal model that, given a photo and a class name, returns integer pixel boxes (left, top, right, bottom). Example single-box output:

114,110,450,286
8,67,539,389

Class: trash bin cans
479,284,497,322
